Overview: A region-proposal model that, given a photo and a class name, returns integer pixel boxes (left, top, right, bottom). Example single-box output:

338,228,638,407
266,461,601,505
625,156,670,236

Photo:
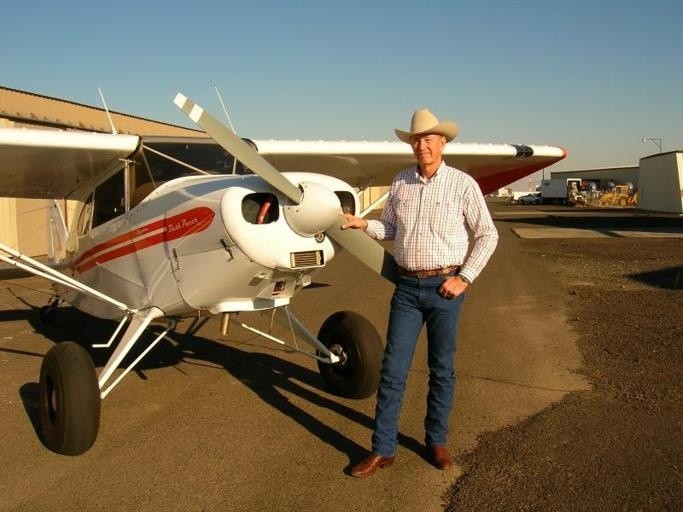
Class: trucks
541,179,581,205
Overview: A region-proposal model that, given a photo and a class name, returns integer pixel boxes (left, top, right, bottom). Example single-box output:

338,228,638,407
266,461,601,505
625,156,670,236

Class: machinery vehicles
601,185,639,207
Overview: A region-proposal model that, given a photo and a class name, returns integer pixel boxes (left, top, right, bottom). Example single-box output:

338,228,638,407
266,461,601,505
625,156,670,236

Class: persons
341,110,499,477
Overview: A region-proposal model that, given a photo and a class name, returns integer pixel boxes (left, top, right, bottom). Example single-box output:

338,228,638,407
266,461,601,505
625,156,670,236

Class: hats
394,109,458,144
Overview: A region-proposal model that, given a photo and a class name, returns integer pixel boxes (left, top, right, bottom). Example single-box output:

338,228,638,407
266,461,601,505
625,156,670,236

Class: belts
398,265,458,279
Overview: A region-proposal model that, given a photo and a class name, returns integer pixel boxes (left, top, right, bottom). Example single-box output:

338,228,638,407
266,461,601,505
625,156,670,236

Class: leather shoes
349,454,393,478
430,443,451,470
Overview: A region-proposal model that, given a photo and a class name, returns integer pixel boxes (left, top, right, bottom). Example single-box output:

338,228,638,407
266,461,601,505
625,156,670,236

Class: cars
519,193,541,205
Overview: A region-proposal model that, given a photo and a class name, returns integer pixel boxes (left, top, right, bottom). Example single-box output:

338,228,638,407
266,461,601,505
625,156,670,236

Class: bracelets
458,274,472,287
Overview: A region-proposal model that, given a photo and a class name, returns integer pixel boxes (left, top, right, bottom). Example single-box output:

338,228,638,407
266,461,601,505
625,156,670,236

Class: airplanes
0,86,567,456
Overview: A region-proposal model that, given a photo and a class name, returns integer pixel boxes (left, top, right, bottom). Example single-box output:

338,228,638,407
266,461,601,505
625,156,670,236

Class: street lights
642,138,662,152
